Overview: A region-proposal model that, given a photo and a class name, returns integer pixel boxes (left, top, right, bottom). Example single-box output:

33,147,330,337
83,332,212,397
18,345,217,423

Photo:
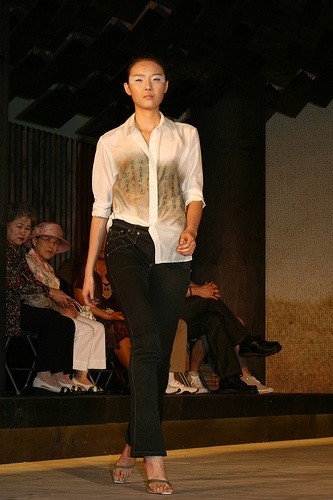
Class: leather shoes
218,376,258,395
72,378,103,392
238,336,282,358
58,380,85,391
32,377,67,393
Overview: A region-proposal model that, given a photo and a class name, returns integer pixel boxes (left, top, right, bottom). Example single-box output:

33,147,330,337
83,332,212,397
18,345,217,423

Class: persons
6,201,86,392
74,234,132,368
81,53,207,494
165,282,282,394
25,224,106,392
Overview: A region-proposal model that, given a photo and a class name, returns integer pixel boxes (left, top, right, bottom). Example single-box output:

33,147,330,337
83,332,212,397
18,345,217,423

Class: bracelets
190,286,192,296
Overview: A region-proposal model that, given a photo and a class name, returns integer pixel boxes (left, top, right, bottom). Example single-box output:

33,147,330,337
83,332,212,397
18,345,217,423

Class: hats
23,222,71,253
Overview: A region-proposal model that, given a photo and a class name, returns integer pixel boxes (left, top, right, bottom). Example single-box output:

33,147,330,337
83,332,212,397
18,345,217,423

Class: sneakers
187,371,209,393
165,385,181,394
240,375,274,394
167,379,200,394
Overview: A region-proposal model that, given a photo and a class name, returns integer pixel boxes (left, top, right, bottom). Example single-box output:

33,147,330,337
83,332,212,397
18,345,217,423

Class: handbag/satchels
70,299,97,321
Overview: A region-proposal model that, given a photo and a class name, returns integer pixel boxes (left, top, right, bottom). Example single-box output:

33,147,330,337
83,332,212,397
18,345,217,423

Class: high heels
112,462,135,484
146,479,174,495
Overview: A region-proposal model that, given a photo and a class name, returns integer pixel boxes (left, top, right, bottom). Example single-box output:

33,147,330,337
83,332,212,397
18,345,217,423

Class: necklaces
98,264,105,278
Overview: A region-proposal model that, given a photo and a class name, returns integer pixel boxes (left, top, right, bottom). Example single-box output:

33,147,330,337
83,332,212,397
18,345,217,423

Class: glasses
39,237,60,247
